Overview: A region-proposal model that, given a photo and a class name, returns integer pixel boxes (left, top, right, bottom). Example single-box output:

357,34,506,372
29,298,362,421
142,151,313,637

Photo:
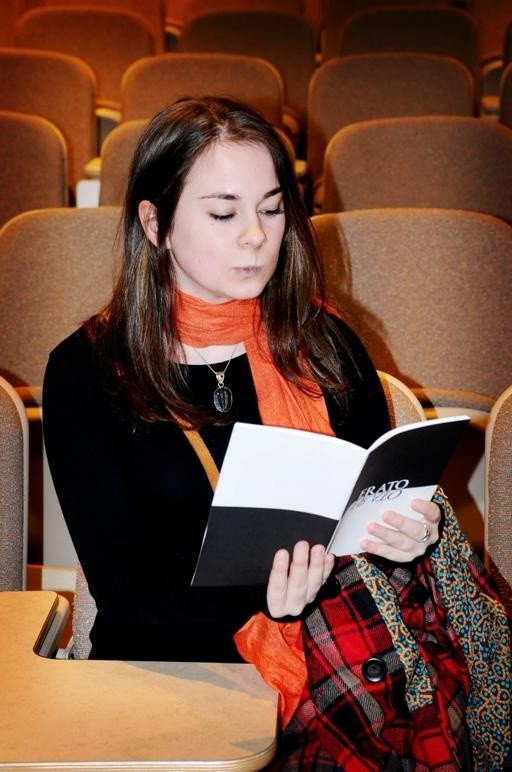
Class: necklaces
190,346,237,415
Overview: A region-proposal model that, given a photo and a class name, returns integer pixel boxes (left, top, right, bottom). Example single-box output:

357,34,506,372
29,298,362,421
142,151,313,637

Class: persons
41,95,512,771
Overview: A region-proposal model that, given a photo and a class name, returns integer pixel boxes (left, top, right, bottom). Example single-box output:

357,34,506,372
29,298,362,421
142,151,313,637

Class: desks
0,590,278,771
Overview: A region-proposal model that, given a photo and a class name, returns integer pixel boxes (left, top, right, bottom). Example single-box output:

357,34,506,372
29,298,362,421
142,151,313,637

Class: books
190,413,471,587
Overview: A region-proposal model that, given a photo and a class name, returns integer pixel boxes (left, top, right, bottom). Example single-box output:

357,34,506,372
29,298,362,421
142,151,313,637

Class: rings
419,524,429,543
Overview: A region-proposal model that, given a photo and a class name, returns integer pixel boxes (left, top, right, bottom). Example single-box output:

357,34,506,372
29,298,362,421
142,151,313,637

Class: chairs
308,53,475,191
0,110,68,229
99,119,296,207
485,386,512,621
410,387,490,425
0,48,97,201
321,116,512,227
0,378,29,591
0,207,125,406
72,370,427,660
308,208,512,412
